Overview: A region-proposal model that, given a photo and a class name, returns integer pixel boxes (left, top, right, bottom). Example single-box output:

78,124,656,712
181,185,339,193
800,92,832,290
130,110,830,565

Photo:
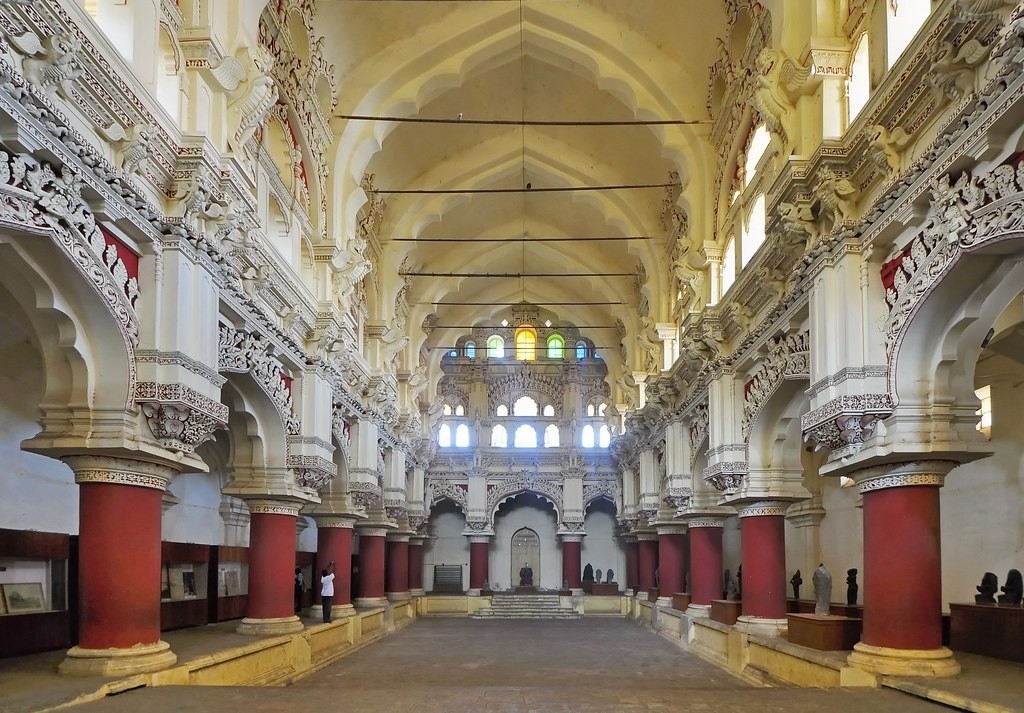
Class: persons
295,568,308,612
320,562,336,624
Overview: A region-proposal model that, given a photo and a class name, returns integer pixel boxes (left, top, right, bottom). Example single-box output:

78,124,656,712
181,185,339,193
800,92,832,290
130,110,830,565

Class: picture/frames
225,571,240,595
1,582,48,614
182,571,196,597
